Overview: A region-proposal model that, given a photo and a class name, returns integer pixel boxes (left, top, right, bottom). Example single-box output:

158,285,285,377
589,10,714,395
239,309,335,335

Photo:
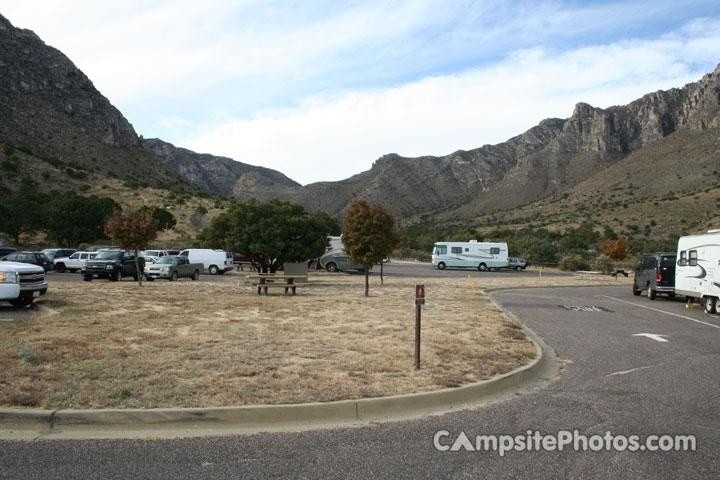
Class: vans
178,248,234,274
41,247,97,274
674,230,720,314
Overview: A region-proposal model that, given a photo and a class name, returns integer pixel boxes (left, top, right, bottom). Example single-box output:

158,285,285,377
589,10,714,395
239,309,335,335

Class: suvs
633,252,676,300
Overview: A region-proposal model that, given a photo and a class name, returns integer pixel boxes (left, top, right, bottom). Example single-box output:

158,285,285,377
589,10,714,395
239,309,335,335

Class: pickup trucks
146,256,204,281
82,249,145,281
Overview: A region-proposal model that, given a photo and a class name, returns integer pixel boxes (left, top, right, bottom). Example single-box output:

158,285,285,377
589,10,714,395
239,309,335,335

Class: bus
269,235,389,271
431,241,510,271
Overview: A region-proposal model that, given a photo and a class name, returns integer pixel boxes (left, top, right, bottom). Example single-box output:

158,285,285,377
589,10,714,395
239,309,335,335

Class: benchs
243,281,312,296
233,261,256,271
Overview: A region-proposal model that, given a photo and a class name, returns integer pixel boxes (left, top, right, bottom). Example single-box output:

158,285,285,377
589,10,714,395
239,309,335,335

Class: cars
508,258,527,271
320,247,369,274
0,247,52,306
141,249,181,262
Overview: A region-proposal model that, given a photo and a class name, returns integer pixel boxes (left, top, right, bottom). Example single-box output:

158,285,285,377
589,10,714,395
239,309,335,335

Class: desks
246,273,307,296
